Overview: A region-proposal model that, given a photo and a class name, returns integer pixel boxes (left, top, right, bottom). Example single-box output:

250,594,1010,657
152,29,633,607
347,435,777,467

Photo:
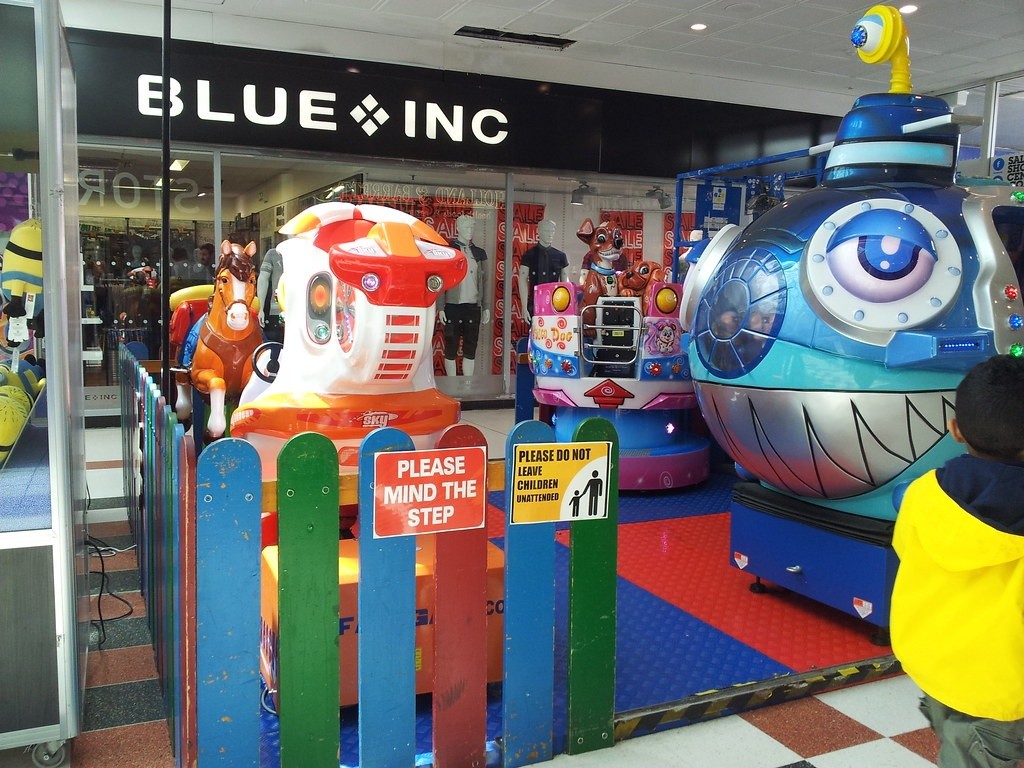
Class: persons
198,243,215,285
579,222,629,285
256,247,285,346
518,220,570,328
436,214,490,376
121,244,211,326
667,230,703,285
888,354,1024,768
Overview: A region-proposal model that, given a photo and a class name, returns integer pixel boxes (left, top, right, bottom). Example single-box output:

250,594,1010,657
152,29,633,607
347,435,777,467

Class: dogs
618,259,665,317
575,217,625,339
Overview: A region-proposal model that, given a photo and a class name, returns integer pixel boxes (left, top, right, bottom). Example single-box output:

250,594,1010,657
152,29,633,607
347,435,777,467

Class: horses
171,238,260,442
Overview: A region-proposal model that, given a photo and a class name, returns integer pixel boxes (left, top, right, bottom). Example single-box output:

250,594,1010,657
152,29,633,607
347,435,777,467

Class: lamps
570,180,597,206
646,185,672,209
151,159,190,188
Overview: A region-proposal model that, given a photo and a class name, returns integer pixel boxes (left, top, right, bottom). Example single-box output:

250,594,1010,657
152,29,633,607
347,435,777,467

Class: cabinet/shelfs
79,253,104,367
